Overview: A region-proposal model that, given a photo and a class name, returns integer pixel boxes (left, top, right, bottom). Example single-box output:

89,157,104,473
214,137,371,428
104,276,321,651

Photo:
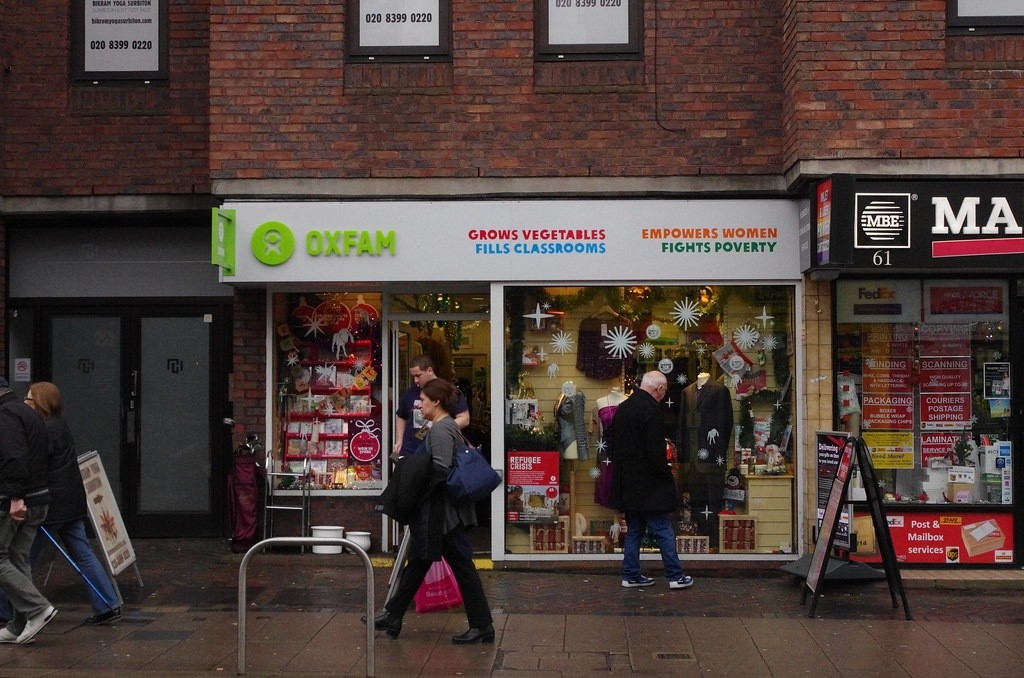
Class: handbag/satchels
412,558,462,614
426,420,504,507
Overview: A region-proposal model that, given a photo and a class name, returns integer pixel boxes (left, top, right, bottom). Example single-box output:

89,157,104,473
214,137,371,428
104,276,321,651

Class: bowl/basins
345,532,372,555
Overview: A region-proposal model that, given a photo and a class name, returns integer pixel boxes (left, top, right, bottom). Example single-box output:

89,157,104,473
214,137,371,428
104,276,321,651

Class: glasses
23,396,34,401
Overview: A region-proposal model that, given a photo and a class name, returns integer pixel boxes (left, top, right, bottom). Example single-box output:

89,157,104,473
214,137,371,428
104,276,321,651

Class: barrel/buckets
311,526,345,554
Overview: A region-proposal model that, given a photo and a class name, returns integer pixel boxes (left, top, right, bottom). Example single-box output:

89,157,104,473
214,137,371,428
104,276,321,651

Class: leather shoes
361,610,403,639
452,623,496,644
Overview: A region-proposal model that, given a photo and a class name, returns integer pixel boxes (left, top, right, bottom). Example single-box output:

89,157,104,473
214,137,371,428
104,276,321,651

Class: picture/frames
585,518,618,551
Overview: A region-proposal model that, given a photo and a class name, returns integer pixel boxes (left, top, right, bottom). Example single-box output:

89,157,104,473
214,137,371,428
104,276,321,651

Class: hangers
580,293,633,327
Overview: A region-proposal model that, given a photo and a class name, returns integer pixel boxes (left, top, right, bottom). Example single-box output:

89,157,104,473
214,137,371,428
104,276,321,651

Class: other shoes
85,607,121,626
669,574,694,588
0,628,35,644
621,575,656,587
16,605,59,644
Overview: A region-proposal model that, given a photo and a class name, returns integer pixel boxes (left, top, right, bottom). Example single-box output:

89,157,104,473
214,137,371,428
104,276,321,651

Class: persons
553,380,591,460
1,379,123,645
590,386,631,522
359,354,494,644
608,371,693,588
681,372,734,553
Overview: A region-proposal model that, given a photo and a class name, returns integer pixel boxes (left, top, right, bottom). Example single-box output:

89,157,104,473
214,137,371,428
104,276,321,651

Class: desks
744,474,796,553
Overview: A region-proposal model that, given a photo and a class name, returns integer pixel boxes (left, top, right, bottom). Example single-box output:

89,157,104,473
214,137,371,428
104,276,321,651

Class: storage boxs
505,397,538,427
529,513,759,554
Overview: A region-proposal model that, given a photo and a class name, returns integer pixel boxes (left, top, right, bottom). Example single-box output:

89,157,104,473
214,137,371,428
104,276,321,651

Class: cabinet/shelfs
262,450,311,555
